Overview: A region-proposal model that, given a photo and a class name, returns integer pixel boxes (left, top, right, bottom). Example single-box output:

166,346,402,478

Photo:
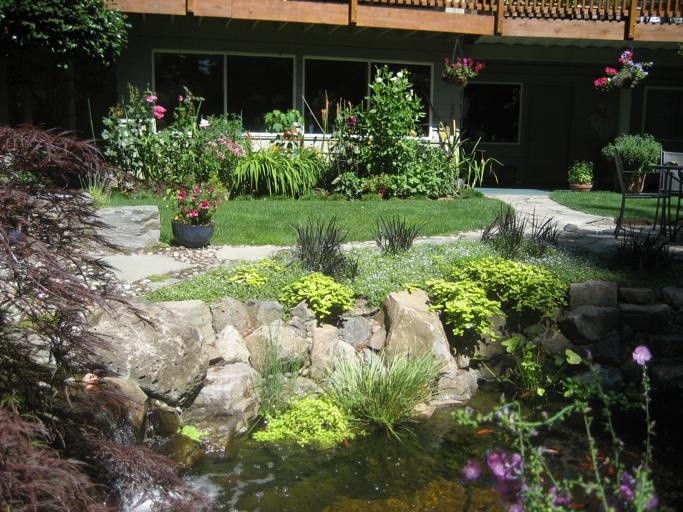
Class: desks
648,164,682,235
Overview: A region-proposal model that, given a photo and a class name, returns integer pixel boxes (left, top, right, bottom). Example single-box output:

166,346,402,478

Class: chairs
612,145,670,241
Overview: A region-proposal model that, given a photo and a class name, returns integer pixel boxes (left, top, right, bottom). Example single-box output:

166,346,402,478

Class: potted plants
600,133,663,194
566,159,594,193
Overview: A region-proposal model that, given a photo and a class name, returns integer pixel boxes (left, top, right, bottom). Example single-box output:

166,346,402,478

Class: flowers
163,173,228,223
434,56,486,81
592,50,654,94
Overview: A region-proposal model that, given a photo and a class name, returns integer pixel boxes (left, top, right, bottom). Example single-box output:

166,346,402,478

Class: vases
170,218,215,248
